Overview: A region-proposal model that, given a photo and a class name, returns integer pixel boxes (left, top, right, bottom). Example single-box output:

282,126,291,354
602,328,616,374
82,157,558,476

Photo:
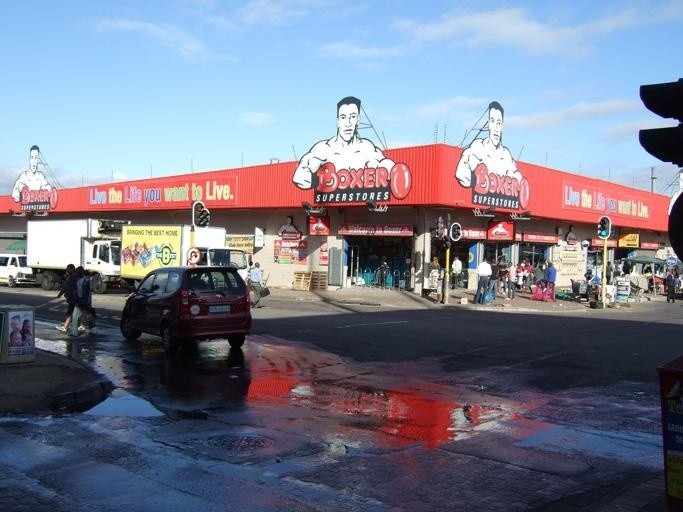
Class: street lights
597,216,611,240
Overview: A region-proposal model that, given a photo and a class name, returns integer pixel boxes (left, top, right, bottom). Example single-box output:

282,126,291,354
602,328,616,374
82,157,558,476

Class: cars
118,265,252,354
0,253,41,289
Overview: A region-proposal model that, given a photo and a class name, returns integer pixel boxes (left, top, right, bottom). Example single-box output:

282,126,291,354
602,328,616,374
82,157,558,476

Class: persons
22,318,31,333
8,142,52,201
9,330,22,348
453,100,527,195
54,262,85,332
247,260,264,309
71,264,97,335
277,215,300,238
9,317,21,331
291,95,394,189
426,254,682,311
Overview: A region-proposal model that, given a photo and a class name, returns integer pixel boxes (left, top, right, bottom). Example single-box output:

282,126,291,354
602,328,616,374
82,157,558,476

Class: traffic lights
634,75,683,167
190,200,204,228
201,209,210,227
442,235,450,249
449,222,461,242
435,215,443,241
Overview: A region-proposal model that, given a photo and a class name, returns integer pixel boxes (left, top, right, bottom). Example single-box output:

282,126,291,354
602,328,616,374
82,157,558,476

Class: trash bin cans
0,304,36,364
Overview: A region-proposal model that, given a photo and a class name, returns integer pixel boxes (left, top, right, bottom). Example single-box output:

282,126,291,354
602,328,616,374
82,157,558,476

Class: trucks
25,217,133,293
118,223,252,294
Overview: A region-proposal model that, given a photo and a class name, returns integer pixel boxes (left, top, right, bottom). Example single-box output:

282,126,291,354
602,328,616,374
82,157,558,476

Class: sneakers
55,326,66,332
77,325,85,330
